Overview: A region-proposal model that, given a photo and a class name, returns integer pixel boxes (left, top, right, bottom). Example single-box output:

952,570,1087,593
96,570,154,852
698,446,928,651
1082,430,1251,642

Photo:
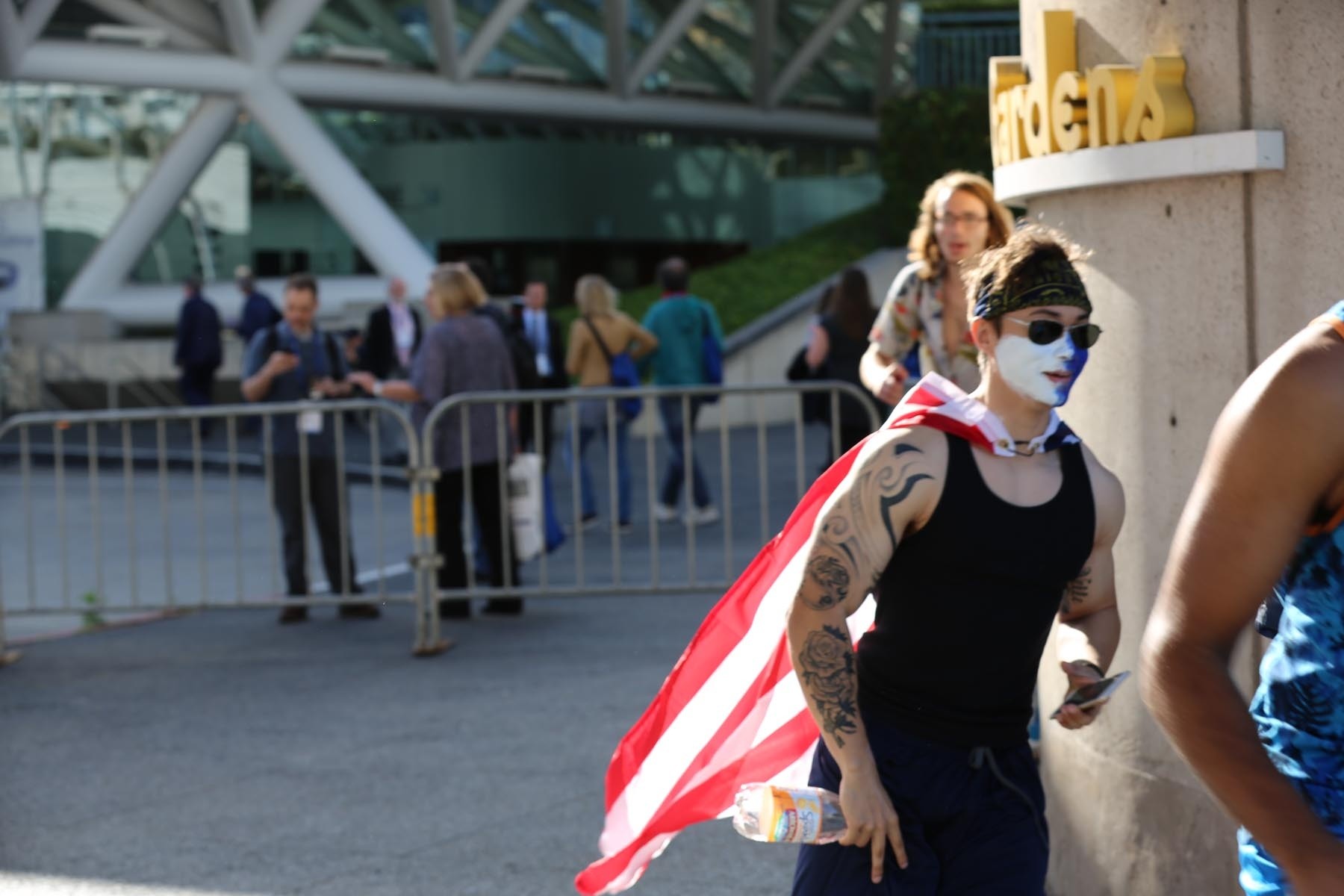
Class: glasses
934,214,989,229
1004,316,1102,351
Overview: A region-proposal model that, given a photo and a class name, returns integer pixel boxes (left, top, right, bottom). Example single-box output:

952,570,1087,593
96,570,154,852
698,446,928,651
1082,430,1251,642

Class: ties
531,311,540,354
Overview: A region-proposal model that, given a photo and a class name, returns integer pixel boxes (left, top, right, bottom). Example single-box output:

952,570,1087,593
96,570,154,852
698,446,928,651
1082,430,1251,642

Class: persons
796,263,886,478
237,271,384,625
1141,296,1344,896
631,258,726,527
559,274,661,533
858,170,1018,403
356,262,528,619
784,224,1127,895
170,273,225,439
219,264,286,434
345,276,567,478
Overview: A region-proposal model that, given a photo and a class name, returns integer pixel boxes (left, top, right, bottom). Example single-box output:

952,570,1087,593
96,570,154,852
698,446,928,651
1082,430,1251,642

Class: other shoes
582,515,599,531
278,607,304,624
438,602,466,618
654,502,678,521
694,507,720,525
340,601,381,621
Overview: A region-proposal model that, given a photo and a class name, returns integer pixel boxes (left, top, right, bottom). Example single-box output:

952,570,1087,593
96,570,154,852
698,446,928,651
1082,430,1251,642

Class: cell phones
1049,668,1132,720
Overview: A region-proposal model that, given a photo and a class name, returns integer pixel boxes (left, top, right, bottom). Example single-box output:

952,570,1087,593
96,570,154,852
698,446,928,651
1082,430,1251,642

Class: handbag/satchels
611,353,644,420
697,305,720,406
508,451,545,564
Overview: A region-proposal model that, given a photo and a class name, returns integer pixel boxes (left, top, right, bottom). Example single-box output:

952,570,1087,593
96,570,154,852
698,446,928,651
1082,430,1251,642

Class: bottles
732,783,848,843
